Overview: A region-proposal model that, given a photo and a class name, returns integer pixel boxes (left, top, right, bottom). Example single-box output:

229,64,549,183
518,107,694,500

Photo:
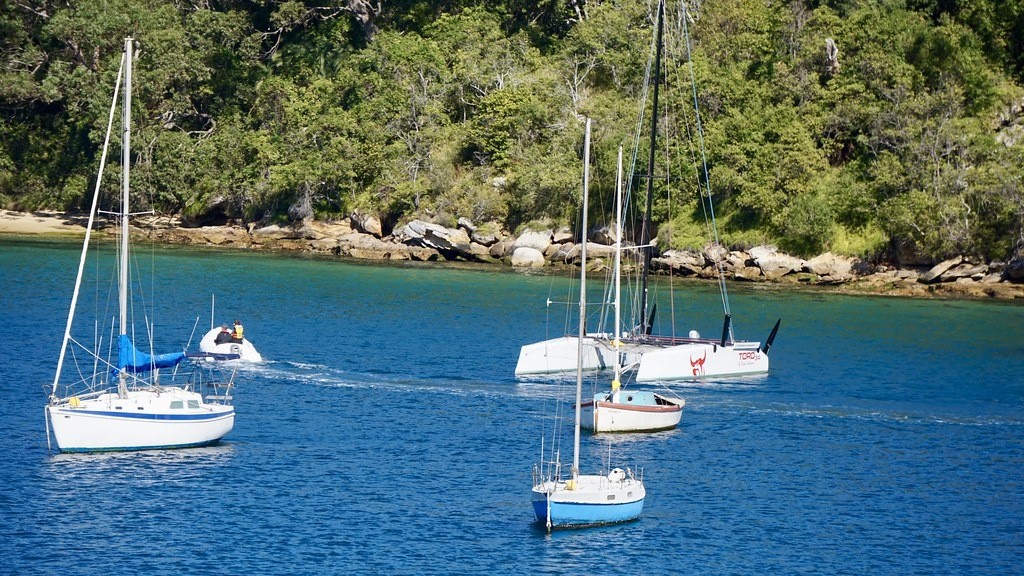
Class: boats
199,323,258,361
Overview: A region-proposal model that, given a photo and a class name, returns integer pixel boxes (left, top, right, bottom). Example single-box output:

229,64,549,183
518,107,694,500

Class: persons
214,323,233,346
230,320,244,344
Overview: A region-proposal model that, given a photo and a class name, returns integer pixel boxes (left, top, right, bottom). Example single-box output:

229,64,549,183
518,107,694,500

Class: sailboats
590,141,689,437
509,0,788,381
530,116,651,531
43,33,239,451
